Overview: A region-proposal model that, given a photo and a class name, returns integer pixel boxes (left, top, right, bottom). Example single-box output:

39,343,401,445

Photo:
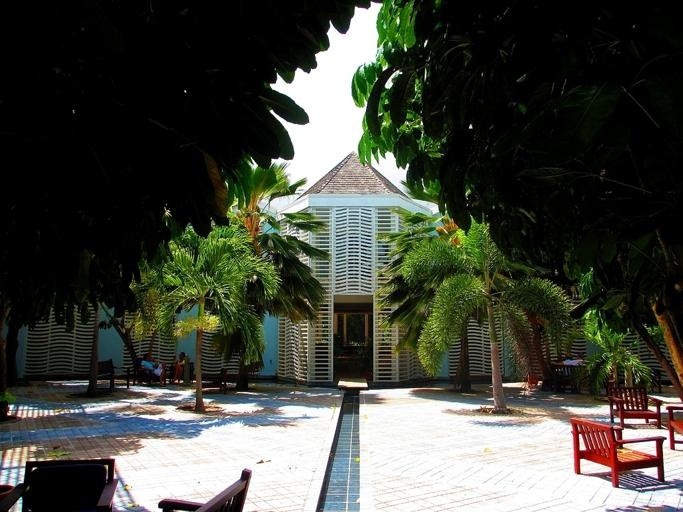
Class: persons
141,353,166,387
168,352,186,385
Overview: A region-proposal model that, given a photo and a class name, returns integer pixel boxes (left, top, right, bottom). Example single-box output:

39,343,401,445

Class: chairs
96,357,194,389
569,385,681,488
0,458,252,512
551,363,663,395
